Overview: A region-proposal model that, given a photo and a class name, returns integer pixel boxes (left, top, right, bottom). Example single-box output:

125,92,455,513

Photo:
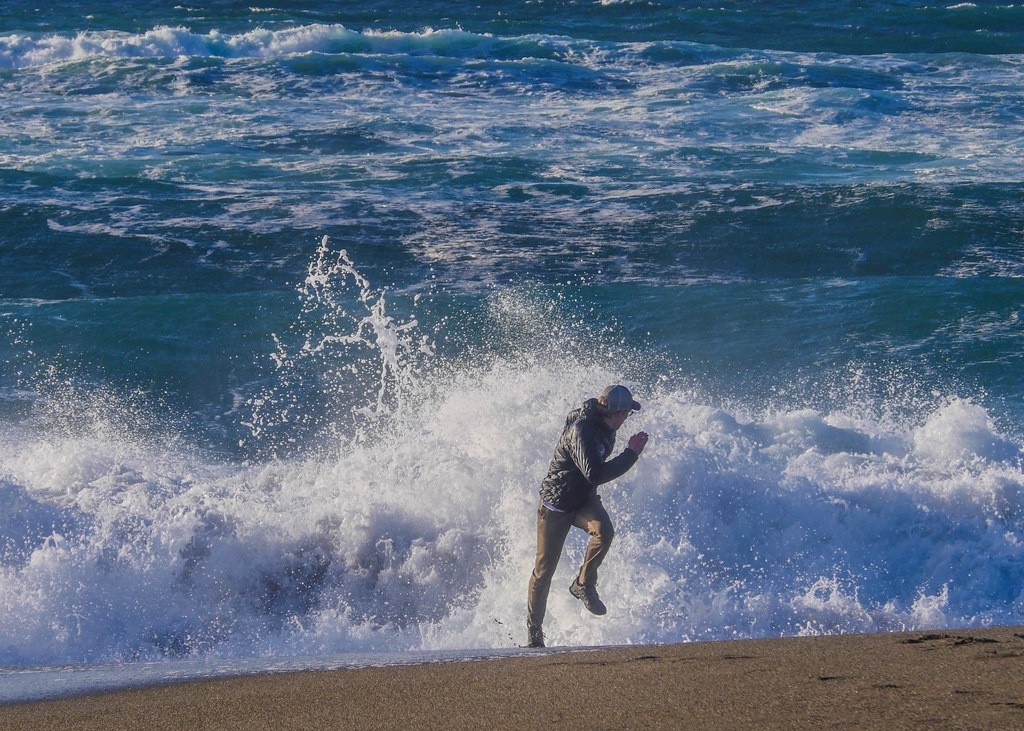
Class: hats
597,385,641,412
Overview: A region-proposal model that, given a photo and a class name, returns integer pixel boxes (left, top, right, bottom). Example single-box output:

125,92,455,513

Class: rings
643,437,645,438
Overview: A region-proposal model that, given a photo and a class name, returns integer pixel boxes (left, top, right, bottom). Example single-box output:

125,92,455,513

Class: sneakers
569,577,607,615
526,622,545,648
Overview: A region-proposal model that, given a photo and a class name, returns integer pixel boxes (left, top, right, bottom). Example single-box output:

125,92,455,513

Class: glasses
624,409,634,416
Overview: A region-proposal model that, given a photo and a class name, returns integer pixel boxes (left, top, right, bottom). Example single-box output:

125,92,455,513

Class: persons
526,385,648,649
